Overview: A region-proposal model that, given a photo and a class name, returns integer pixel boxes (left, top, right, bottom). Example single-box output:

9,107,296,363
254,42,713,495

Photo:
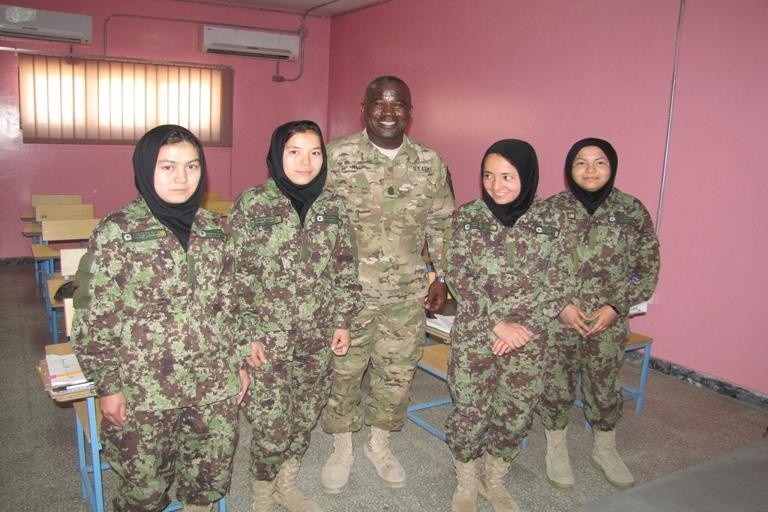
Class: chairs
19,191,123,340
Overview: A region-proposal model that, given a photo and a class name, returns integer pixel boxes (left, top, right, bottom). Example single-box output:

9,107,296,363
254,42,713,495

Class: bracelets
606,303,621,315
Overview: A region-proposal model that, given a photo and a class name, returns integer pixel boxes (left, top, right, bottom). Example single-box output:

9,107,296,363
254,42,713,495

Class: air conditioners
0,4,94,46
198,25,300,64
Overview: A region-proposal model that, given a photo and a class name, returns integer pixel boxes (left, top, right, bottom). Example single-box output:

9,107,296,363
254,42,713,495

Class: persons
69,124,252,511
225,120,365,511
319,74,457,494
538,138,662,490
442,138,577,511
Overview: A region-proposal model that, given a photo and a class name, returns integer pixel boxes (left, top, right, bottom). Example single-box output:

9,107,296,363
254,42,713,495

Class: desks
33,343,228,512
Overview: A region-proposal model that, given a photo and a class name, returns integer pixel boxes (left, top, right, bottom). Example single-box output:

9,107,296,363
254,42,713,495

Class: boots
451,457,480,511
590,429,635,488
251,482,276,511
545,428,575,491
479,452,518,512
322,432,353,493
183,504,212,512
275,458,321,511
363,425,407,488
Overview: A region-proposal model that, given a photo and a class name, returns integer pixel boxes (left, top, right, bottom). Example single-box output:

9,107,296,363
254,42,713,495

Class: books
36,347,96,400
425,312,456,335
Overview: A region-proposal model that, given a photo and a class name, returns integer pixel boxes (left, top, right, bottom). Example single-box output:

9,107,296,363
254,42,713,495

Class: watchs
436,274,446,285
